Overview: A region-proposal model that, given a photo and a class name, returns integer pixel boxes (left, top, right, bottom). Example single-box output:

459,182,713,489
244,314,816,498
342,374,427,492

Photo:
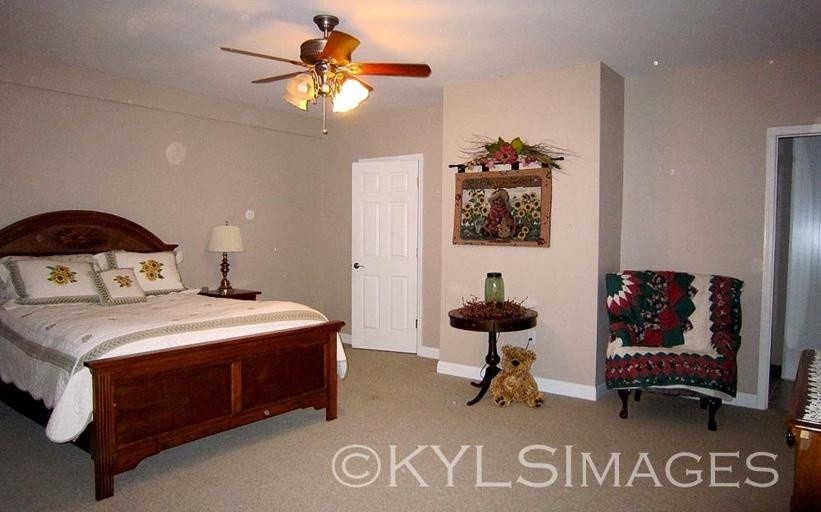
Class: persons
479,189,521,240
490,345,545,408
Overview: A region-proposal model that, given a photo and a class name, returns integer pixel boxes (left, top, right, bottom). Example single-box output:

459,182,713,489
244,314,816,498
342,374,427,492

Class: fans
218,14,432,97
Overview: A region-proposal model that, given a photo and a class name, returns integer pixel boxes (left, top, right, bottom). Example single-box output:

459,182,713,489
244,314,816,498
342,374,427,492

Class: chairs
602,269,746,434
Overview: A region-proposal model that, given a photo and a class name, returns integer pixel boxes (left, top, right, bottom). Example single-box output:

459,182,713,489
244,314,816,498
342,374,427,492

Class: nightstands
200,288,261,305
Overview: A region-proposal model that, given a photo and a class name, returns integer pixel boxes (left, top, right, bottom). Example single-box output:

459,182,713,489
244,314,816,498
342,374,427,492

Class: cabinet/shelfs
784,344,820,510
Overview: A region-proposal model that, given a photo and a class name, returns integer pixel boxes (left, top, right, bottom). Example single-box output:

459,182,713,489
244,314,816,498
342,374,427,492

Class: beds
0,211,348,503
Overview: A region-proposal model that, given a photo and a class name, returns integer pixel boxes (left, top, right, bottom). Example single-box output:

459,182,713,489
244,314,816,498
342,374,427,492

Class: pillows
90,265,149,304
91,249,191,295
0,252,105,305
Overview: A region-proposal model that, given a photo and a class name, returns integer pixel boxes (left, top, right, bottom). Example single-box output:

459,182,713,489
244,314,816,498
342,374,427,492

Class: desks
446,305,537,406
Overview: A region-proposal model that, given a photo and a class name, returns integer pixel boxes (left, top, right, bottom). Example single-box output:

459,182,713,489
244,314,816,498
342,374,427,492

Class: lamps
205,218,245,295
279,56,369,115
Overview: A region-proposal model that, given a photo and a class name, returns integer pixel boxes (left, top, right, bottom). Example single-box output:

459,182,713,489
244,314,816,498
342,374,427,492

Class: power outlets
527,329,537,346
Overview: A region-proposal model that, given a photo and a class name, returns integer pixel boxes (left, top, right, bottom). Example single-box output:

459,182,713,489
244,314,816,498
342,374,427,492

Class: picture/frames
450,170,552,248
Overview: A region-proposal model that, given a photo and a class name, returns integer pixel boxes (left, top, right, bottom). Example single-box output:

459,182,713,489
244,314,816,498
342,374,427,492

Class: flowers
461,187,540,242
466,133,539,170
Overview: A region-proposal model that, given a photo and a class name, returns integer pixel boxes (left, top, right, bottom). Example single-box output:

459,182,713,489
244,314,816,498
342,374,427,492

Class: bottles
485,272,506,304
